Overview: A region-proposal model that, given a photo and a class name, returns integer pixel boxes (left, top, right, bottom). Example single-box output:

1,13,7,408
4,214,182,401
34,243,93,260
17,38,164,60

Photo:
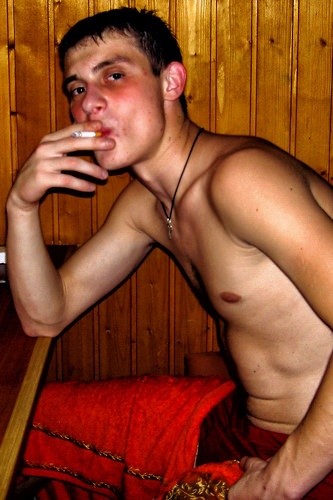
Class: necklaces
160,127,205,240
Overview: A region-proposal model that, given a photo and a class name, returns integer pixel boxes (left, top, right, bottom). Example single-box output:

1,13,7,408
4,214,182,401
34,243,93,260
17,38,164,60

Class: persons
6,6,333,500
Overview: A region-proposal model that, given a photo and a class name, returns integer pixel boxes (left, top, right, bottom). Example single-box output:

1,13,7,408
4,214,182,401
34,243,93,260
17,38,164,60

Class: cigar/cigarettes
73,131,102,137
